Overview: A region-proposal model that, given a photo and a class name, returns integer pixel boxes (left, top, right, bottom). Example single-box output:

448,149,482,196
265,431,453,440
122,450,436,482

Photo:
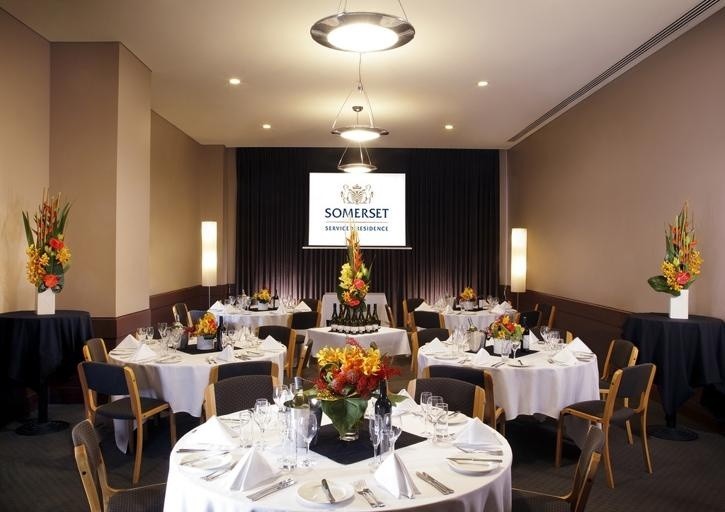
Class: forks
548,358,566,366
209,461,238,480
458,358,468,363
456,445,503,455
461,359,470,364
355,481,377,508
212,359,217,364
201,462,231,480
360,480,385,507
207,358,211,364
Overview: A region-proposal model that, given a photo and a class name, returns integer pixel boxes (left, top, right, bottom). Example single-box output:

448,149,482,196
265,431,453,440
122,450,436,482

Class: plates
434,351,463,360
448,453,499,474
240,350,264,357
434,410,467,424
158,355,182,364
298,479,354,505
509,359,532,367
182,451,232,468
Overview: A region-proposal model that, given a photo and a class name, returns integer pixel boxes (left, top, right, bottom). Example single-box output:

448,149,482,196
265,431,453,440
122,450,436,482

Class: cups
437,404,449,439
239,411,252,448
291,383,297,398
500,342,509,360
282,430,298,470
557,339,564,354
380,430,394,465
160,340,169,357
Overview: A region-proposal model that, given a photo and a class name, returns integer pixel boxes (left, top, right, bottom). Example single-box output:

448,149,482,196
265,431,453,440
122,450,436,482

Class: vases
35,286,55,316
338,408,361,441
464,299,474,311
669,289,688,320
491,338,512,355
197,335,215,350
257,300,269,310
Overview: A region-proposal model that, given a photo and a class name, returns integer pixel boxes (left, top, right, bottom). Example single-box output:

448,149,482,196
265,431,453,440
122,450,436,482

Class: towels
424,337,448,353
492,304,503,314
116,334,139,349
133,343,157,361
227,446,279,492
444,305,455,313
565,337,592,353
192,414,238,450
277,304,286,313
373,453,419,500
415,302,431,310
453,417,501,445
225,305,237,314
296,301,311,310
552,347,577,364
473,347,495,364
258,335,284,351
391,389,418,417
216,345,234,362
501,301,512,310
526,330,539,346
209,300,224,311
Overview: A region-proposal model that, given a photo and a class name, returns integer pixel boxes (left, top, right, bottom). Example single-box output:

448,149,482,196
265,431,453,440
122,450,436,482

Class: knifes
322,479,335,503
449,410,461,417
247,351,261,355
491,361,502,368
495,363,506,368
576,354,592,362
248,479,291,498
181,450,229,466
416,472,448,495
235,354,245,360
242,354,250,360
160,356,177,362
518,360,524,366
446,458,502,462
253,481,297,501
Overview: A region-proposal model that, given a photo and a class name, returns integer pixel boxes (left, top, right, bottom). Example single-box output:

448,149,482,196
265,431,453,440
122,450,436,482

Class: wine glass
370,415,383,465
163,328,171,350
255,398,270,453
170,330,181,353
272,385,288,413
459,294,472,316
144,327,154,343
426,397,444,441
159,322,167,340
136,326,146,345
422,392,431,435
220,325,258,354
486,295,500,312
282,295,299,313
510,334,521,360
301,414,317,465
540,326,559,353
385,413,402,454
229,295,251,310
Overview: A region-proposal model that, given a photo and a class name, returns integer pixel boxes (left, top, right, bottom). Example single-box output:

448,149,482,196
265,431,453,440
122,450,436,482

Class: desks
320,291,389,327
626,313,724,442
0,309,90,437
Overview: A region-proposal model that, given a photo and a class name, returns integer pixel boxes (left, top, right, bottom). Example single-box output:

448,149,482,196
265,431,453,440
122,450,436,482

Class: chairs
513,311,541,334
188,309,206,327
172,301,193,330
422,365,505,438
288,312,320,370
403,297,426,330
408,312,447,376
203,375,279,422
199,361,280,423
406,377,483,427
511,424,605,512
384,304,411,367
77,361,176,486
83,335,111,426
411,328,449,378
72,419,166,512
597,338,639,446
556,362,654,489
533,304,554,330
299,297,321,323
282,338,314,389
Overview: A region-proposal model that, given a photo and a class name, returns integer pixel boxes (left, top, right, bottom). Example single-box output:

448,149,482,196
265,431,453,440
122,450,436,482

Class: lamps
330,54,389,143
201,220,218,309
336,107,379,174
510,227,528,311
310,0,415,55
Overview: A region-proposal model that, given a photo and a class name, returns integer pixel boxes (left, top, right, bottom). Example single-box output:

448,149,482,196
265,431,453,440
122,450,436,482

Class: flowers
19,185,75,295
335,217,374,310
459,286,477,301
283,339,407,436
249,288,272,304
191,312,217,336
487,313,522,342
646,200,704,297
